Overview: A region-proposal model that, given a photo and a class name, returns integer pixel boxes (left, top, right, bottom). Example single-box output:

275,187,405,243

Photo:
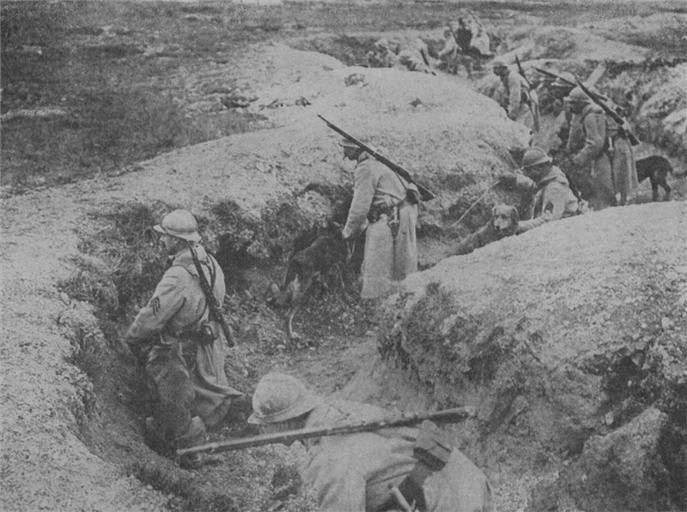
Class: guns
318,114,436,201
574,72,640,145
531,66,607,100
184,238,233,347
176,405,478,455
516,54,525,78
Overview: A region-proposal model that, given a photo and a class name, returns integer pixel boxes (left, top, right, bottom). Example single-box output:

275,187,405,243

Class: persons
335,133,419,300
564,84,641,205
507,146,582,236
365,7,496,83
487,55,541,132
242,369,500,512
116,208,243,443
549,71,619,156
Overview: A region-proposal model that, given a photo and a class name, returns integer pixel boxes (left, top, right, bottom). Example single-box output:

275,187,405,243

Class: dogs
548,149,617,210
270,204,383,340
636,156,673,202
446,203,519,257
135,339,225,469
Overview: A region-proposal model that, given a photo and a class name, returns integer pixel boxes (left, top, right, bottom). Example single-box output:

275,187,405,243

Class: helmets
153,209,198,241
562,86,589,103
338,129,368,148
522,146,552,168
246,371,318,424
551,71,576,87
490,55,507,68
